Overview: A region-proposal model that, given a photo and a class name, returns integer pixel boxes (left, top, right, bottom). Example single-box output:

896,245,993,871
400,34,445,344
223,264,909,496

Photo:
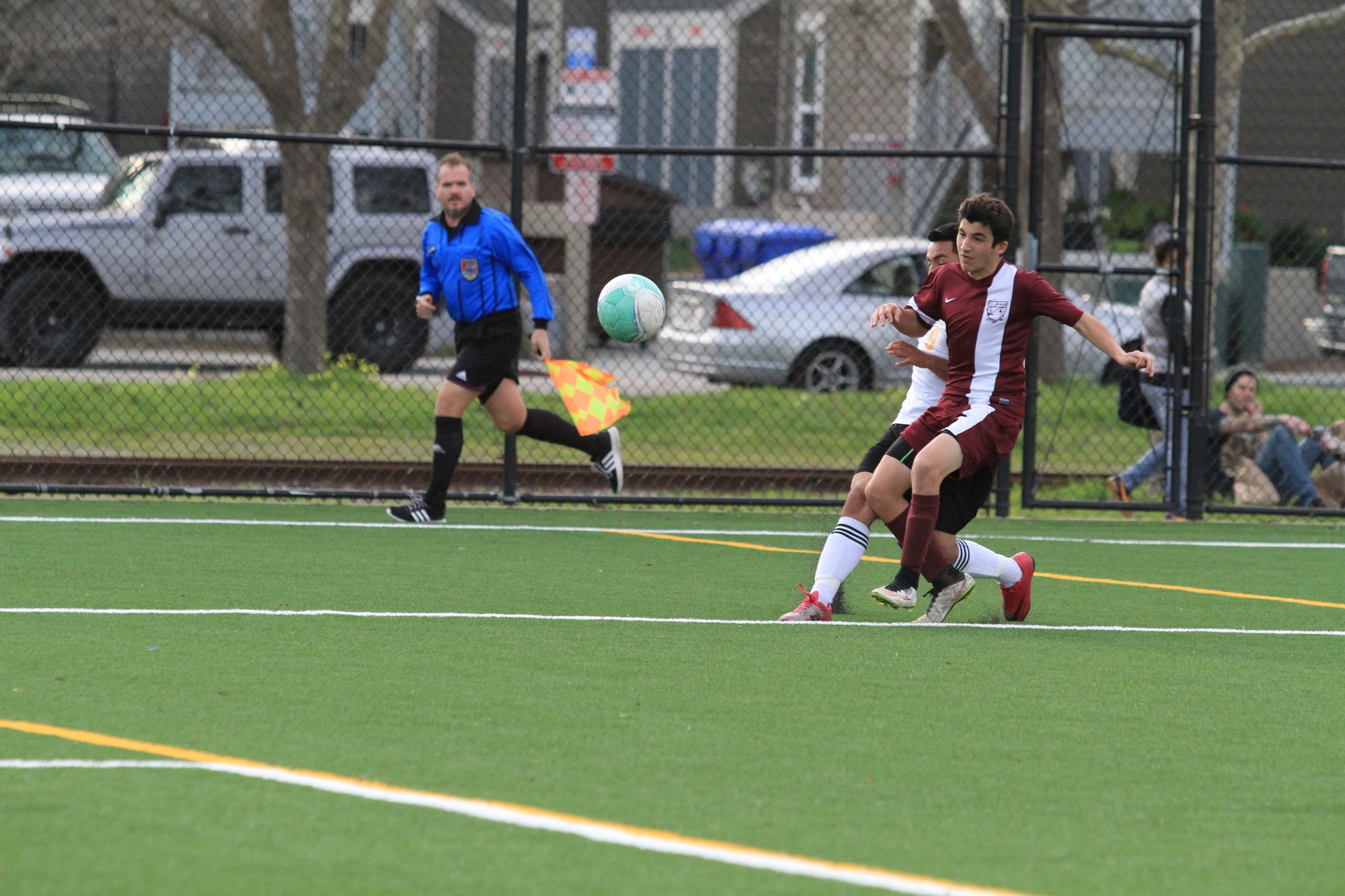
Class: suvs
0,141,454,372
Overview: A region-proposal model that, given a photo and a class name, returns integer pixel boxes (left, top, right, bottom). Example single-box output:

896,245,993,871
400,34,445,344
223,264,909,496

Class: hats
1222,362,1258,394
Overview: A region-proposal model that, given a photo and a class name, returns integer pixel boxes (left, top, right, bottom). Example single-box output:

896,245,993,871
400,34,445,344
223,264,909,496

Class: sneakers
778,597,832,622
385,499,447,523
1164,512,1191,521
1104,475,1131,517
871,577,916,612
999,551,1035,622
591,426,624,494
911,571,975,624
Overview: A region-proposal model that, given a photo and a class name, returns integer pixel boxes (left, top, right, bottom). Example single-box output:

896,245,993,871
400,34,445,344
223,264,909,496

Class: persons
384,151,624,523
1206,369,1339,514
768,193,1204,625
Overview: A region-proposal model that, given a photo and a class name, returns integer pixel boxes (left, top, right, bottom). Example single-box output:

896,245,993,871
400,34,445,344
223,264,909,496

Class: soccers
597,272,667,344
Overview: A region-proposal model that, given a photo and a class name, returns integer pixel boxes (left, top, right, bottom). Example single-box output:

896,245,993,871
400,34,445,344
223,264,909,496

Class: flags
544,360,631,436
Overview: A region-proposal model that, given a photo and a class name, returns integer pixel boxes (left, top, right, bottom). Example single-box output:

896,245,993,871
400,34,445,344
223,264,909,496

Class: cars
646,236,1153,386
0,91,124,214
1313,241,1344,359
